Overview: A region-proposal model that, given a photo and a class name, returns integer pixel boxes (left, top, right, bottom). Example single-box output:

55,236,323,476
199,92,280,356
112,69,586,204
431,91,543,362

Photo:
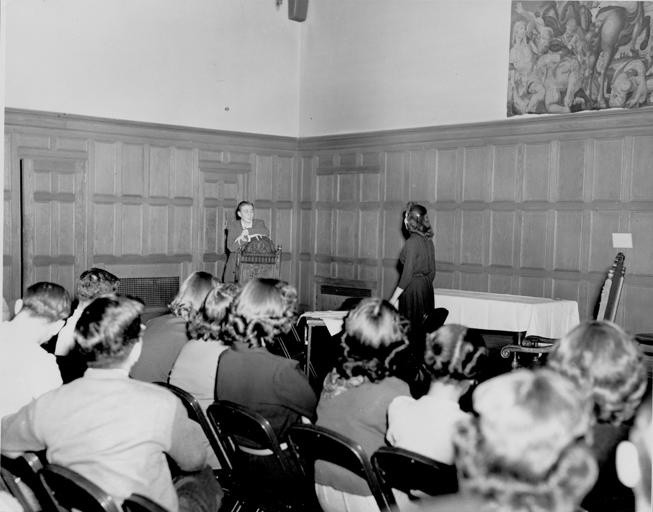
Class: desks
430,287,580,370
303,309,351,383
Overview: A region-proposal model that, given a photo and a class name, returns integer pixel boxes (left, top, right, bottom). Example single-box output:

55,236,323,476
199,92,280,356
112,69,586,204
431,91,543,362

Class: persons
221,201,270,284
506,1,652,114
1,265,652,512
387,200,436,361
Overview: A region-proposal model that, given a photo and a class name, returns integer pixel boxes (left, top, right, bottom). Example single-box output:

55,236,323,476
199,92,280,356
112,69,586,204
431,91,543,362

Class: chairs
499,251,626,371
